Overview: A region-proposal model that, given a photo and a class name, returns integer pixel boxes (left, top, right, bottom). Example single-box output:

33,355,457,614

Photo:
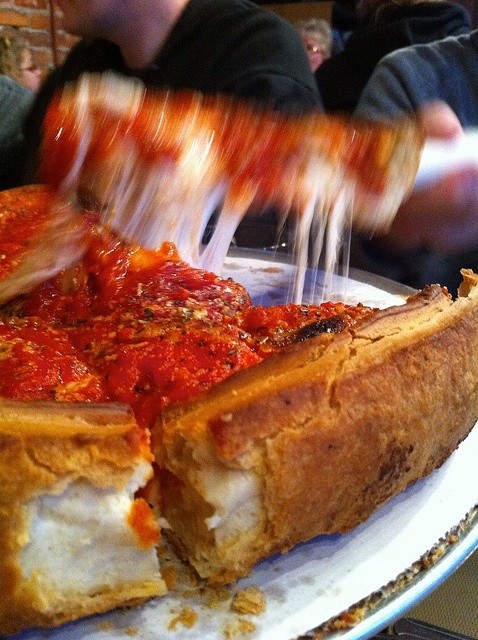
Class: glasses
306,44,324,54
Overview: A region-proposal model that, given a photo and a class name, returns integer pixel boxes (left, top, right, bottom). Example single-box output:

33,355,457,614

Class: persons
25,0,327,171
321,0,476,69
0,77,37,188
294,20,336,70
348,32,477,249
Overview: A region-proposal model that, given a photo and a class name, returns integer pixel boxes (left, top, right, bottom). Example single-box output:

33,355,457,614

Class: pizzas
40,73,431,229
